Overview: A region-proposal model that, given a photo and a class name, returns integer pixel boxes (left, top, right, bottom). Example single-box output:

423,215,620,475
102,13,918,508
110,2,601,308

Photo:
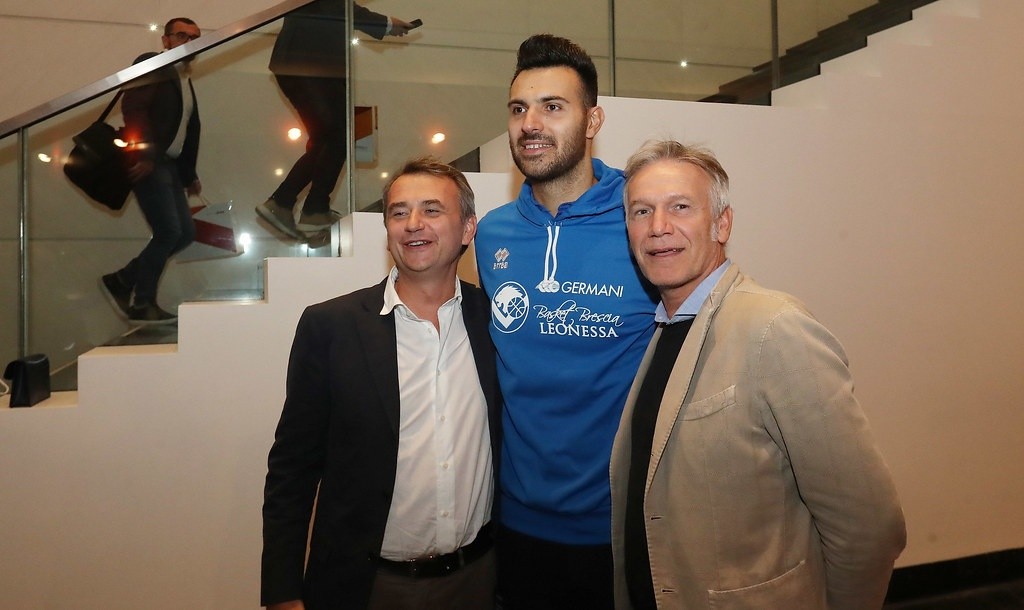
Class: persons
262,157,502,610
252,0,417,241
461,34,666,610
97,16,204,326
618,143,905,610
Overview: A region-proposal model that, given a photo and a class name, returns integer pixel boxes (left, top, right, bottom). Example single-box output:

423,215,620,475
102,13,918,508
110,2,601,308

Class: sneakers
255,197,300,239
99,273,132,321
129,302,176,326
297,208,339,231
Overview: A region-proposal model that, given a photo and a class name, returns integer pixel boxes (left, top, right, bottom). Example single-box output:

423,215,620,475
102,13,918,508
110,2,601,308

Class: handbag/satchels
174,193,245,264
61,119,138,214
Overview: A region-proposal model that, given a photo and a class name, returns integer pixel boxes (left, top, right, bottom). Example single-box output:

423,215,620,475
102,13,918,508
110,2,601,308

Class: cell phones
409,19,423,30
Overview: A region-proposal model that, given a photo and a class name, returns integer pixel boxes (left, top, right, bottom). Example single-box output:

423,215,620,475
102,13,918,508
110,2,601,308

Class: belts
377,537,486,578
